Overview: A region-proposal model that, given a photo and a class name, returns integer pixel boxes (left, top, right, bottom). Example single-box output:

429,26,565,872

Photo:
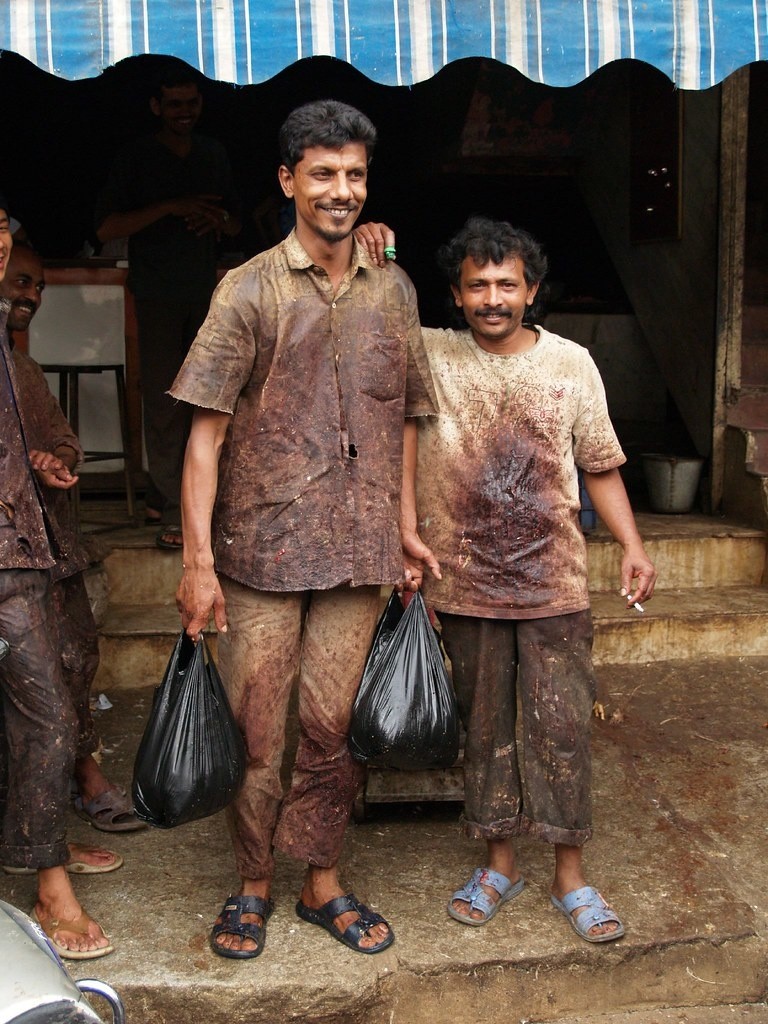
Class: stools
37,363,136,532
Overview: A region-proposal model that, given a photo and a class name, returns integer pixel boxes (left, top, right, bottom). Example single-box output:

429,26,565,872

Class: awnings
1,0,767,91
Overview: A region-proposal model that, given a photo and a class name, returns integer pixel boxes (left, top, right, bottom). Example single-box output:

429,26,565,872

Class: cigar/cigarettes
627,595,645,613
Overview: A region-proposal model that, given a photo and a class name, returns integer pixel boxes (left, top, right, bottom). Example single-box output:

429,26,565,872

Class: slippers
296,893,395,954
211,896,274,958
447,867,524,926
156,526,183,548
550,885,625,942
74,789,147,831
2,840,124,874
145,518,161,526
30,907,114,959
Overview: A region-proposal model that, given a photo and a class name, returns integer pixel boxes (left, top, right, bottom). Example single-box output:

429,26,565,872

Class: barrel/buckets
643,453,703,515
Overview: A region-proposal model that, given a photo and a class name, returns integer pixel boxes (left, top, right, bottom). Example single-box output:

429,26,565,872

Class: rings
197,212,204,221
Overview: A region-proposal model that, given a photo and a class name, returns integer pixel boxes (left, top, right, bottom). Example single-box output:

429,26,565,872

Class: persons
0,198,116,959
0,238,150,831
352,221,659,942
172,98,441,959
95,63,241,548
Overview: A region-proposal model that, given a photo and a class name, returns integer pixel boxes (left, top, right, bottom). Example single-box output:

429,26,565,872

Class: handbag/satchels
347,583,460,773
130,629,247,829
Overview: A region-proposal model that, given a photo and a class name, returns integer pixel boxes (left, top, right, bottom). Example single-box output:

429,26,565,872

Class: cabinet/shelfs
13,268,150,496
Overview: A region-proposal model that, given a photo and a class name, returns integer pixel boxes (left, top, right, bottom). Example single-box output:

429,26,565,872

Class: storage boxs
576,471,597,534
